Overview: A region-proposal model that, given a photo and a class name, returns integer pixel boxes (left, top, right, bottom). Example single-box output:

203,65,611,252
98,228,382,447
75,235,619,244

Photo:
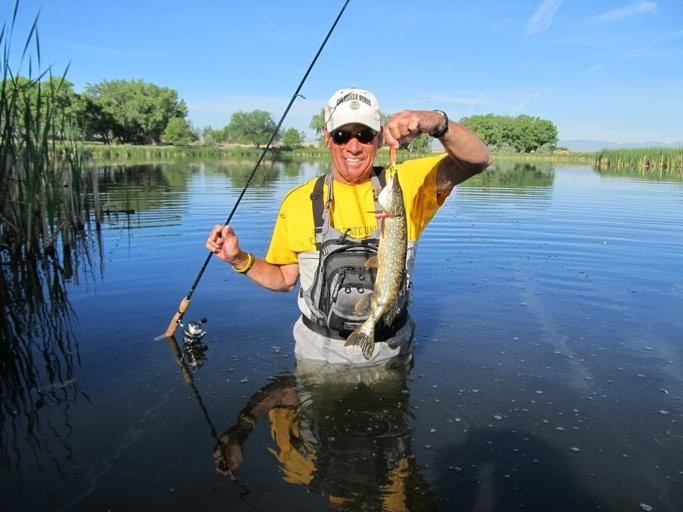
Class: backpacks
302,167,412,334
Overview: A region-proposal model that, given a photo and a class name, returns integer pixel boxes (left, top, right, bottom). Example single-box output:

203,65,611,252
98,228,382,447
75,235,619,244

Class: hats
324,87,384,135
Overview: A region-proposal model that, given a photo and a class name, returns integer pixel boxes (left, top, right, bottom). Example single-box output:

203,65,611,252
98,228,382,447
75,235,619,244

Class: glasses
328,129,376,145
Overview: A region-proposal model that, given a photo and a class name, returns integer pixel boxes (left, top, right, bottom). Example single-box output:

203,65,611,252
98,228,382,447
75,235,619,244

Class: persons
214,366,436,511
204,85,494,367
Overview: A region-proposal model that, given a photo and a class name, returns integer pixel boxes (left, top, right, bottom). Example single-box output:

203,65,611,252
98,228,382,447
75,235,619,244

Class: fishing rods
167,0,351,343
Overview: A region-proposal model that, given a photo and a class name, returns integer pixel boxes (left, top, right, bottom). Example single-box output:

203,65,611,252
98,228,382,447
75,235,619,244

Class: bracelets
231,252,255,275
238,410,258,428
428,109,449,138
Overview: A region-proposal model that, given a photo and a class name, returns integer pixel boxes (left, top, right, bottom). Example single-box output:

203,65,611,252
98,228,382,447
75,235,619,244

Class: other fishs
342,167,410,361
